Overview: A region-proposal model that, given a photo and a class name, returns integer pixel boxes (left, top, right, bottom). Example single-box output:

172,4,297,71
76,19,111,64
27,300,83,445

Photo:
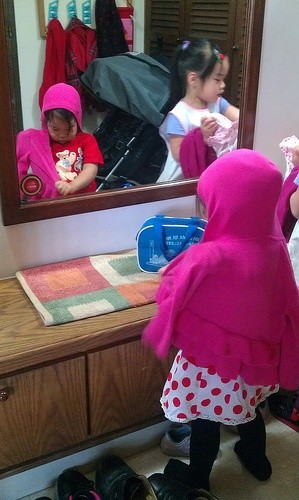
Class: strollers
78,50,170,189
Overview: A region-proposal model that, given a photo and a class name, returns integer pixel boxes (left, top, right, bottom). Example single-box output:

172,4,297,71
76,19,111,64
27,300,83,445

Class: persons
16,83,105,202
154,38,240,181
277,135,299,227
141,149,299,489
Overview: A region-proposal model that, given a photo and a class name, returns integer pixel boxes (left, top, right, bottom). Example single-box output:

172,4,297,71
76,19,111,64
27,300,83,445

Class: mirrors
0,0,266,227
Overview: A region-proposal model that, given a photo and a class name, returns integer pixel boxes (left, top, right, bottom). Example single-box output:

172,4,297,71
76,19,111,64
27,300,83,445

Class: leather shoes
146,473,220,500
95,454,158,500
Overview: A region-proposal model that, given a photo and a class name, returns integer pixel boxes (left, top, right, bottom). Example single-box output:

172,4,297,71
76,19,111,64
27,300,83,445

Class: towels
141,149,299,388
38,18,67,112
180,126,217,179
15,247,161,327
16,83,84,202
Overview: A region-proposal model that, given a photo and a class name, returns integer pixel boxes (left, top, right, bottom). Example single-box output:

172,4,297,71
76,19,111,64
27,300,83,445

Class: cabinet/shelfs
0,248,161,479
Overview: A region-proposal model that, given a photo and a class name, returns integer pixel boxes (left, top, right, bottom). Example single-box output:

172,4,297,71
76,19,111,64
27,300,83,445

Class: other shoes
160,426,192,456
57,469,100,500
267,393,299,433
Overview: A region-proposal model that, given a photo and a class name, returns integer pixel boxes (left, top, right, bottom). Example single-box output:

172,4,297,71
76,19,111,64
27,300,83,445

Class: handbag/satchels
136,214,208,274
